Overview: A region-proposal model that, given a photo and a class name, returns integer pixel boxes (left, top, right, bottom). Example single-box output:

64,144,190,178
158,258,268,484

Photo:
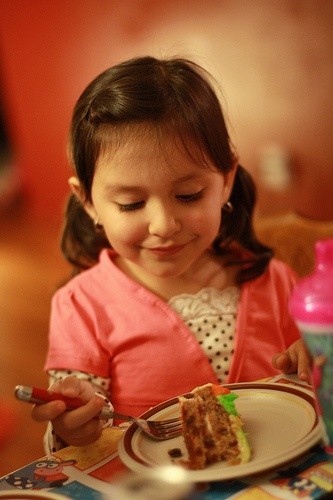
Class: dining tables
0,373,333,500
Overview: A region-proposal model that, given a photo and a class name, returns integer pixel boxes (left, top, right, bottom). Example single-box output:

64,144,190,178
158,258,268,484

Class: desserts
179,382,250,471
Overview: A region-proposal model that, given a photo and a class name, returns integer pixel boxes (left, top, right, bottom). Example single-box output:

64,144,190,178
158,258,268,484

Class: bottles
287,239,333,451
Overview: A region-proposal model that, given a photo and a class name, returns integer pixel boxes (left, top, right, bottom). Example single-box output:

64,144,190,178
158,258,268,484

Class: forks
13,382,187,443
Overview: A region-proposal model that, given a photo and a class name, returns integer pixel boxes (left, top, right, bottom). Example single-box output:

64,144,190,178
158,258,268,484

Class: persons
30,56,313,459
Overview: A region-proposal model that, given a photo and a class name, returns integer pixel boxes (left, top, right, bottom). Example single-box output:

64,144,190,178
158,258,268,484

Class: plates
117,379,328,485
